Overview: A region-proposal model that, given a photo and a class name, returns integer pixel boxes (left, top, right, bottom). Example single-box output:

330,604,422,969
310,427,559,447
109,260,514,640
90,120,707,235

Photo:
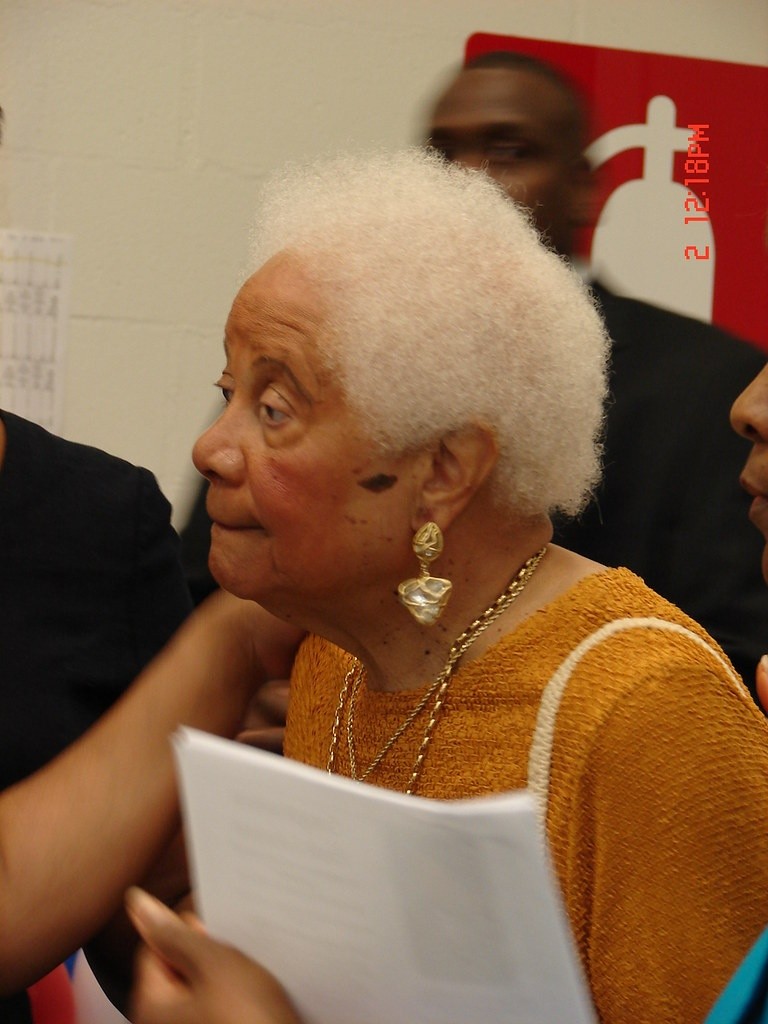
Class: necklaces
347,548,546,783
323,548,546,796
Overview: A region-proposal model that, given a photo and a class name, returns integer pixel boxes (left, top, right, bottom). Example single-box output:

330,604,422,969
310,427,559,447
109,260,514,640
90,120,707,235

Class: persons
705,363,768,1023
429,53,768,715
2,145,768,1023
